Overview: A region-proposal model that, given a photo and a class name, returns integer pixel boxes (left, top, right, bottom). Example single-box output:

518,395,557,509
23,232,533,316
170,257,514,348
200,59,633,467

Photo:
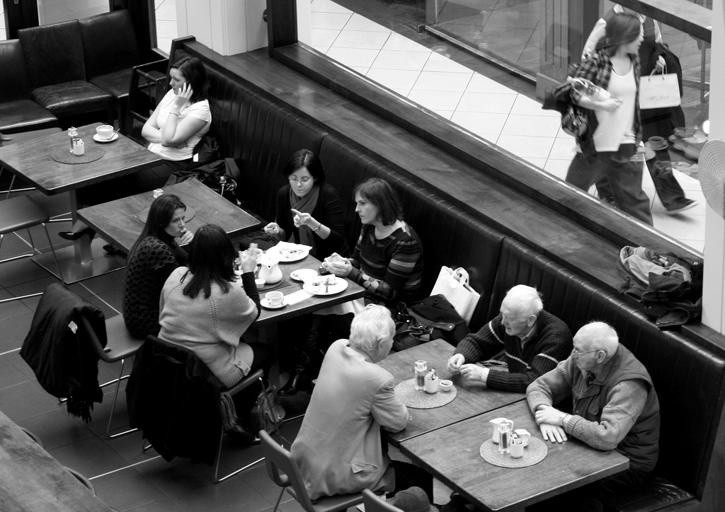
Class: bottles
500,422,511,453
414,360,428,392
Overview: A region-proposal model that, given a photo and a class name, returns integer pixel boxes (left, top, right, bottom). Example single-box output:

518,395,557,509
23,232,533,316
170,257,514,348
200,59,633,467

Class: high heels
58,226,95,241
102,244,125,255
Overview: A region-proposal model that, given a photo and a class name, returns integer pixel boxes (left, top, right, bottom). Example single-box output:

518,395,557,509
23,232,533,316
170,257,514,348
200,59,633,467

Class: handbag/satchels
431,266,480,325
639,74,681,110
257,386,286,436
392,306,431,351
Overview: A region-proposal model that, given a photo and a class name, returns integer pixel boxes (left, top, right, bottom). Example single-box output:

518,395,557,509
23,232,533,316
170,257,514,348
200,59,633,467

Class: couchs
142,47,724,512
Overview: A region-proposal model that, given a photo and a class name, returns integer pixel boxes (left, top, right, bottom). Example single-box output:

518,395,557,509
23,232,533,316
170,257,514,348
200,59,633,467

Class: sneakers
666,197,694,215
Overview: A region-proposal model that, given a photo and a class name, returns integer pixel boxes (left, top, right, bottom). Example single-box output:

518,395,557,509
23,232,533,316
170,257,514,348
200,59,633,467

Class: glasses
575,349,600,358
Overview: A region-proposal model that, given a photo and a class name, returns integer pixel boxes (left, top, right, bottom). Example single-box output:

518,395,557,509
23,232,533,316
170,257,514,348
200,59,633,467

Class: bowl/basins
440,380,453,391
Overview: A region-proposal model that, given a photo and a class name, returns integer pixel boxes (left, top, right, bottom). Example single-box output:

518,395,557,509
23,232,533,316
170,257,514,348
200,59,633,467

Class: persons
581,4,698,216
264,149,350,374
116,192,195,338
276,176,423,397
58,55,212,248
525,322,660,498
564,13,654,232
446,283,573,504
158,223,272,447
286,304,436,502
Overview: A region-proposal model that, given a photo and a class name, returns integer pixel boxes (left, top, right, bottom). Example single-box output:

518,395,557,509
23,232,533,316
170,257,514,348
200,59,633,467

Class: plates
267,245,309,263
302,275,348,297
93,133,118,142
290,268,318,282
260,296,289,309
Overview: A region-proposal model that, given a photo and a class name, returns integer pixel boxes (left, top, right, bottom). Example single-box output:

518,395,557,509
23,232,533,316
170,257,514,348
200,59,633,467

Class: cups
73,143,85,156
255,279,265,288
424,376,439,393
265,291,283,305
514,429,531,447
256,249,264,259
96,125,113,140
509,444,523,457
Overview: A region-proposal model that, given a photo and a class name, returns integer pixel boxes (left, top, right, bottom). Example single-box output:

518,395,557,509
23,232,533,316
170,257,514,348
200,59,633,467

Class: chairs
256,428,407,512
1,8,155,135
72,304,283,483
0,194,65,304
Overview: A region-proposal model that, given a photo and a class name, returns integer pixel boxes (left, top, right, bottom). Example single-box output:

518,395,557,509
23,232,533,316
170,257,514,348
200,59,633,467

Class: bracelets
168,111,179,117
312,224,322,232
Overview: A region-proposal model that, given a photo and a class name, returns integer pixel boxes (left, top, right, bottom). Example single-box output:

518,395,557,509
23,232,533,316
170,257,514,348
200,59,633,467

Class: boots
277,313,324,396
